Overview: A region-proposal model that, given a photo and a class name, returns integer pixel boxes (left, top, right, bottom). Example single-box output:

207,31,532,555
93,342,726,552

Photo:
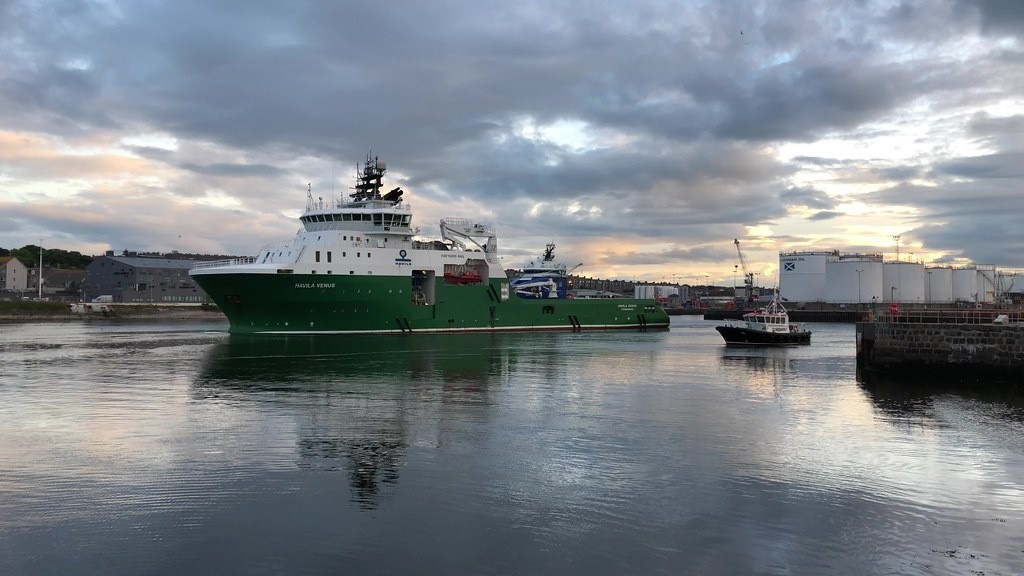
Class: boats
443,269,483,284
714,277,812,346
186,148,671,336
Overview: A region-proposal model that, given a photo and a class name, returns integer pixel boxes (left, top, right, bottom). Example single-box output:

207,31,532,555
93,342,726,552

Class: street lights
748,273,753,310
855,270,865,302
755,273,761,308
893,236,901,302
705,275,709,303
927,272,933,308
39,238,45,301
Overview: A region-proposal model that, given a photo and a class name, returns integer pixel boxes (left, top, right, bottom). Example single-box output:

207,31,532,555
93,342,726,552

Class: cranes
733,238,756,287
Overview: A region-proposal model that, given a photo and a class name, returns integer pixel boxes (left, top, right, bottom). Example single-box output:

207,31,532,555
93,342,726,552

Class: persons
889,302,899,322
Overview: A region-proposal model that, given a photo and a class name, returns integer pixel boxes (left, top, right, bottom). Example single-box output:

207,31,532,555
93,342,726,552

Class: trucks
92,295,113,303
